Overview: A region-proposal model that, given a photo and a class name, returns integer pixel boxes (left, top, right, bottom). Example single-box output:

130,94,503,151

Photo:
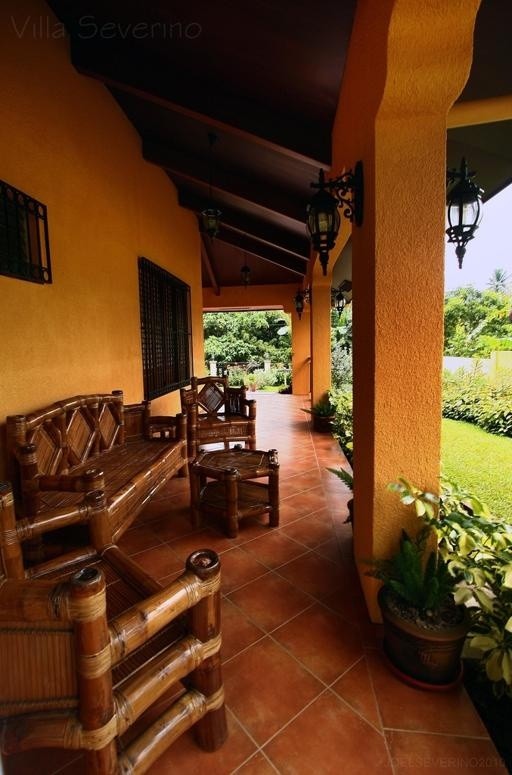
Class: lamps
303,157,363,278
328,280,352,319
199,131,223,244
440,155,491,271
239,251,251,290
291,285,312,321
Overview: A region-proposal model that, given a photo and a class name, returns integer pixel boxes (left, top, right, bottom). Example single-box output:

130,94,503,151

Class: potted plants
298,389,339,433
361,521,474,692
